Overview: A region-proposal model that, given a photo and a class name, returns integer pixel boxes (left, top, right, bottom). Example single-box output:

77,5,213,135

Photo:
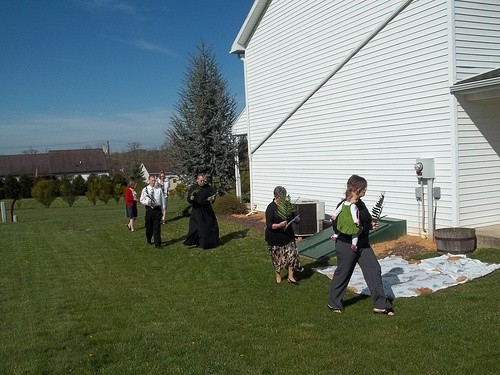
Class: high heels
276,279,282,284
287,278,299,285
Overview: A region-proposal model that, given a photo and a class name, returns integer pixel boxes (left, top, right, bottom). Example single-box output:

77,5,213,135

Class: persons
331,189,364,252
327,175,395,315
125,179,138,232
184,173,219,250
266,186,304,284
157,173,170,223
139,174,165,249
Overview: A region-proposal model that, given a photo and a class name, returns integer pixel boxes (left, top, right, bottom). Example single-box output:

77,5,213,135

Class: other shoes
351,245,357,252
332,237,336,241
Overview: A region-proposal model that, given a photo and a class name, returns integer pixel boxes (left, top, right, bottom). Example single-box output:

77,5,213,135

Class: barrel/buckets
435,228,477,254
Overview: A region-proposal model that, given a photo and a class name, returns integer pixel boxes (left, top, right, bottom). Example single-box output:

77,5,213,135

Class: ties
151,191,155,209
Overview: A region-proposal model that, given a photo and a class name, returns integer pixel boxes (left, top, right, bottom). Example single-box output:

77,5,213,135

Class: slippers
327,305,341,313
373,308,395,316
